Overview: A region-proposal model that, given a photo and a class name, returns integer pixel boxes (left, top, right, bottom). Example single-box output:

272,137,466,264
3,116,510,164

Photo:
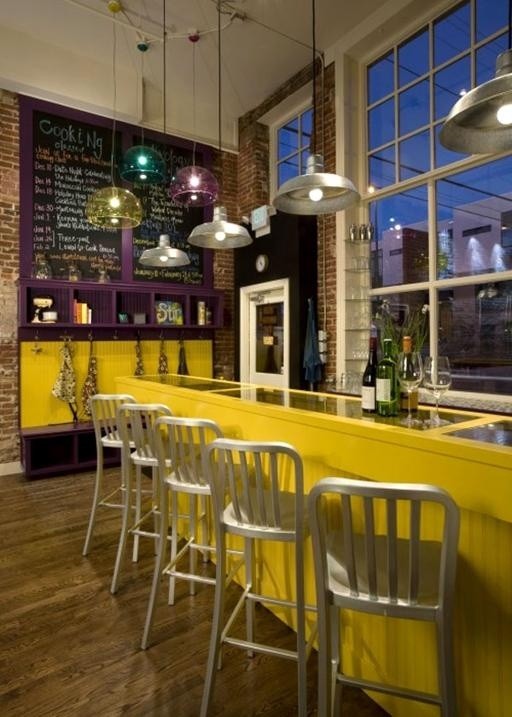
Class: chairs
203,438,327,715
306,476,464,717
89,394,257,671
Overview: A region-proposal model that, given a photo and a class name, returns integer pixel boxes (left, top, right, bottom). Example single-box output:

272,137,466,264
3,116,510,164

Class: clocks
255,254,269,273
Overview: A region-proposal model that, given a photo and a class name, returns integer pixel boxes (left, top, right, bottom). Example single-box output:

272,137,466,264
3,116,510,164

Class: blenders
32,295,59,323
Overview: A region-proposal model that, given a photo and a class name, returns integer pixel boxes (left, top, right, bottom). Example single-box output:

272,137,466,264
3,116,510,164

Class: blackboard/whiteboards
17,93,213,289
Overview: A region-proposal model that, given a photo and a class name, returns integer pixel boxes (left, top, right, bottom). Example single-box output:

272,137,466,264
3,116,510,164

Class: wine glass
423,356,453,425
395,351,424,424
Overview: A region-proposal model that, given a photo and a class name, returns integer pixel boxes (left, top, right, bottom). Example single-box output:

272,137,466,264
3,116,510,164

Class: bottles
398,336,420,414
362,337,378,413
350,223,374,239
31,258,110,281
375,339,401,416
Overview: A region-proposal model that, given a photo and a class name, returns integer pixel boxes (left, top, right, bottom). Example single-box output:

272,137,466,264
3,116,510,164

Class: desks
115,373,512,717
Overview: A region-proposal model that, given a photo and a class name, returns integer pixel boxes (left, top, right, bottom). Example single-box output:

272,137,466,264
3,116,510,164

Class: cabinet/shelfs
14,277,224,481
345,239,373,361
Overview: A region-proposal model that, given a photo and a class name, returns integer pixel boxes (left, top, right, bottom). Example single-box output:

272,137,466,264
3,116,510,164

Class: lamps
272,0,362,216
85,0,254,267
439,0,512,155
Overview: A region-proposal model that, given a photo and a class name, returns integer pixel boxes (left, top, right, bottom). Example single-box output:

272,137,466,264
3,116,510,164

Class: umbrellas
303,298,323,391
